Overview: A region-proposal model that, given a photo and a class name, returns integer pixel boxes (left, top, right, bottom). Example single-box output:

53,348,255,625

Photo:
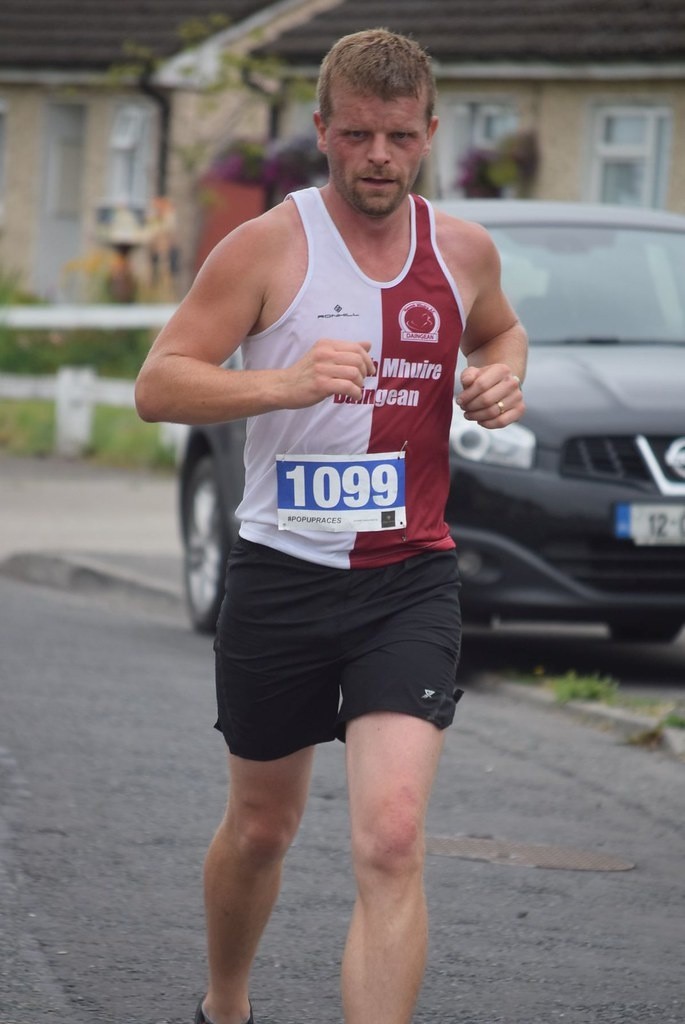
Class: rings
497,401,504,414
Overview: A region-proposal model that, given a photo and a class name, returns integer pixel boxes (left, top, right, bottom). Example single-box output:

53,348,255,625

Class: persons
135,28,528,1024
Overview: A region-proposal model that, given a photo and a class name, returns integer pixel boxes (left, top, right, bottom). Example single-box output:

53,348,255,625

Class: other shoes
194,992,255,1023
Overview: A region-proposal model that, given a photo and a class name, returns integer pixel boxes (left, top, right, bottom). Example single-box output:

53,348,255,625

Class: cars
178,199,685,670
